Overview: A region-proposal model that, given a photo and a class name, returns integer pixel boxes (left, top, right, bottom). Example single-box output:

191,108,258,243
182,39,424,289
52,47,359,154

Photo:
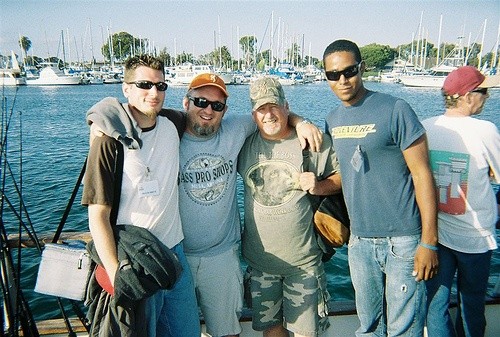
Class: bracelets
419,243,437,251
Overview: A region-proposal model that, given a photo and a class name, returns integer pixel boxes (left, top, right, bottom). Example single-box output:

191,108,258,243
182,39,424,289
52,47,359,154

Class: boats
5,12,338,86
170,65,234,86
0,66,24,86
379,11,500,88
104,72,121,83
29,60,81,84
401,72,500,87
82,70,103,84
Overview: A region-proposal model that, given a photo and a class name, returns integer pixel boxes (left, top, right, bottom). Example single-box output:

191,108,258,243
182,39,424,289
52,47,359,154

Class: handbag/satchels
33,238,92,300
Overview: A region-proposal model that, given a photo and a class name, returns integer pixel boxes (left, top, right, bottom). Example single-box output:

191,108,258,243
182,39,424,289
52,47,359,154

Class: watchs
296,117,312,129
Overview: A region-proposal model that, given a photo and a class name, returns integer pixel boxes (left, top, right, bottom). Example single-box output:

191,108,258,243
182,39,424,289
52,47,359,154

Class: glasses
325,59,363,80
188,96,226,111
471,87,488,94
127,80,168,90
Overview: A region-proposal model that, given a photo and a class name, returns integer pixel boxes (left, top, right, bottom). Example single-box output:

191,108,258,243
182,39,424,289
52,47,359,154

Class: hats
250,77,285,110
188,73,229,96
442,66,500,98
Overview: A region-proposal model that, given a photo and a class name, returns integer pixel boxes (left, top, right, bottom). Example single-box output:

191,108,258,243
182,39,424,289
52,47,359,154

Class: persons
325,39,438,337
237,75,341,337
419,65,500,337
89,74,324,337
82,54,202,337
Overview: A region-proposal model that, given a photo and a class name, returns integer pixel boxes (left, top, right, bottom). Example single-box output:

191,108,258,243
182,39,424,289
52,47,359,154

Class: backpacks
302,129,350,246
